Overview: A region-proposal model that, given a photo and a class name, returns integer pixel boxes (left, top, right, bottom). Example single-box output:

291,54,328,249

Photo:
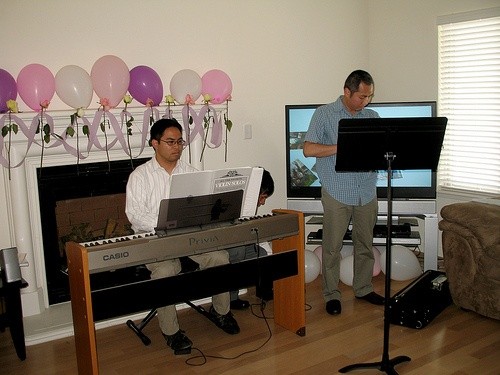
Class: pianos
64,209,306,375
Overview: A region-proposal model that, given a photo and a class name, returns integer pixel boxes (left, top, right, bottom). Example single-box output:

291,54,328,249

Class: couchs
438,199,500,323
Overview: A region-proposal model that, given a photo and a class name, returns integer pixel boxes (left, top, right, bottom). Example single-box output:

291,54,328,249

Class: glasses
160,139,185,146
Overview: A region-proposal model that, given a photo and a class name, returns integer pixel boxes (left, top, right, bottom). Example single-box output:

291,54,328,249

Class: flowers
2,94,233,181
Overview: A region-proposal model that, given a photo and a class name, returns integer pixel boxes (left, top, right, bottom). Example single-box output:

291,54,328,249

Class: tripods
334,117,448,375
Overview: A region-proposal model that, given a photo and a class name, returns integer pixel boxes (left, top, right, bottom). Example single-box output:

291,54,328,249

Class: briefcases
390,269,452,330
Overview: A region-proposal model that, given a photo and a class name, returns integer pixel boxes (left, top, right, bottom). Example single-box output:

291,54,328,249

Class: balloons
0,69,17,114
90,55,130,108
170,69,202,104
16,63,55,110
128,65,163,107
201,69,232,104
305,245,382,285
54,65,93,109
380,244,425,280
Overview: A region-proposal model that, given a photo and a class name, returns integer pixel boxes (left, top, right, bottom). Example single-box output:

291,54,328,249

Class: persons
125,119,240,350
303,70,385,315
226,169,274,309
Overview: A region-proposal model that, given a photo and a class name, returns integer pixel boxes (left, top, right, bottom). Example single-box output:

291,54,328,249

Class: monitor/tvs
285,101,438,199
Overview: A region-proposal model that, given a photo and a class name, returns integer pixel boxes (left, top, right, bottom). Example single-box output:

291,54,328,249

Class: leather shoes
326,299,342,315
230,298,250,310
163,330,193,351
355,292,385,306
210,305,240,334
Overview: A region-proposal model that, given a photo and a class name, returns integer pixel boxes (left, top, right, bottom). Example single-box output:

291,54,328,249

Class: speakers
0,247,21,283
